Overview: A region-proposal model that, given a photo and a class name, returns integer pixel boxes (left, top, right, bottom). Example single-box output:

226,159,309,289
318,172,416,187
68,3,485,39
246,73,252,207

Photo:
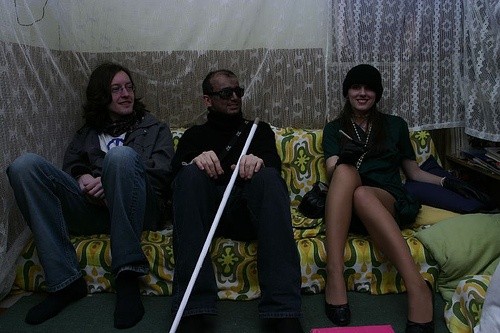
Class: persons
171,69,305,333
322,64,488,333
6,63,175,329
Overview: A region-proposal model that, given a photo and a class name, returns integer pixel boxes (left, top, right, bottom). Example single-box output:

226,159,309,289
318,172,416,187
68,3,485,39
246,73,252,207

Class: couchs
11,123,460,303
413,212,500,333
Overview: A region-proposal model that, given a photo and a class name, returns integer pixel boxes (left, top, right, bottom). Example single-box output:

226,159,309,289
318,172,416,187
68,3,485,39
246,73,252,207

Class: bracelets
440,177,446,186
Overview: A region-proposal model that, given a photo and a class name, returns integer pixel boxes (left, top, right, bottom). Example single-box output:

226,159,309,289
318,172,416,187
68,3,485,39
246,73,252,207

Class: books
473,147,500,174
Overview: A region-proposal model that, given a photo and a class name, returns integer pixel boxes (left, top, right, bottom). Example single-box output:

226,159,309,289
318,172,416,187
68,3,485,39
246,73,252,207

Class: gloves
441,177,491,203
336,149,367,167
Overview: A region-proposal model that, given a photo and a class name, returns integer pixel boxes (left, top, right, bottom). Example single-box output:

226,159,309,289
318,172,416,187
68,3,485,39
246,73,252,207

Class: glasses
110,83,136,94
207,87,245,100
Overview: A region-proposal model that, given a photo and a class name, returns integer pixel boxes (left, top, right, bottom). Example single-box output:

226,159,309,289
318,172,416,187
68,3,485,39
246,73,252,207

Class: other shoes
404,280,436,333
324,279,351,326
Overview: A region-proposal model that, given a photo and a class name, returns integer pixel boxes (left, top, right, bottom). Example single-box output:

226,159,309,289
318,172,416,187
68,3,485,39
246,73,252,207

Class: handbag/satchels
297,180,329,218
